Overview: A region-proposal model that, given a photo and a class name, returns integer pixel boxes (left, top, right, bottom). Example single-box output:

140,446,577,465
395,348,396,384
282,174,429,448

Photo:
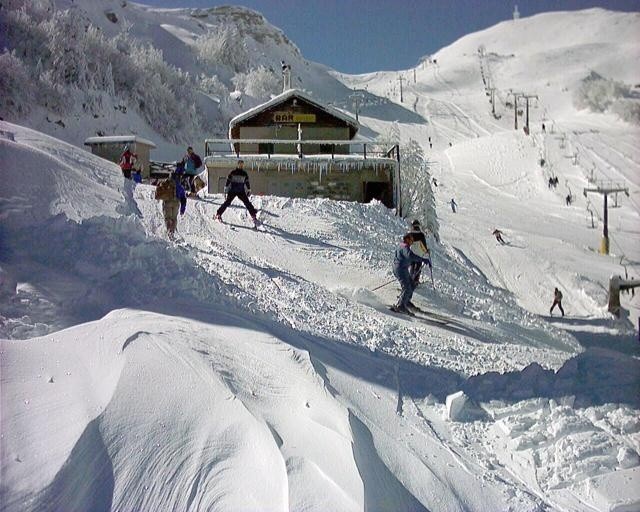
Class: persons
447,199,457,212
121,146,137,180
493,229,505,244
162,173,186,241
216,160,256,219
550,287,564,316
393,235,429,313
182,147,202,197
407,220,428,285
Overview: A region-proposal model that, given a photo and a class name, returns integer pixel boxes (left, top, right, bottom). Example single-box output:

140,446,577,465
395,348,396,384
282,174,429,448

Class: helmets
412,220,420,226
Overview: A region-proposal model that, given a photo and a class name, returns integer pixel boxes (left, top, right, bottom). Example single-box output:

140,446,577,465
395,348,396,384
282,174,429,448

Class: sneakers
251,213,258,224
216,212,223,221
396,300,418,314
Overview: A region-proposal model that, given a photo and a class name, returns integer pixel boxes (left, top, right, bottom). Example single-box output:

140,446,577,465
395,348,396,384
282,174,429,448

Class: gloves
224,188,229,200
246,189,251,197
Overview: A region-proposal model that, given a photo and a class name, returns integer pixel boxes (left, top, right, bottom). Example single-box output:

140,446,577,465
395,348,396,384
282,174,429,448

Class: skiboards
211,210,259,230
387,304,451,324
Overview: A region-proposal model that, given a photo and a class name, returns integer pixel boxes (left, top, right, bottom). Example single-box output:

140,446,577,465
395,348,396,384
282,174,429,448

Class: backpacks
154,180,177,201
